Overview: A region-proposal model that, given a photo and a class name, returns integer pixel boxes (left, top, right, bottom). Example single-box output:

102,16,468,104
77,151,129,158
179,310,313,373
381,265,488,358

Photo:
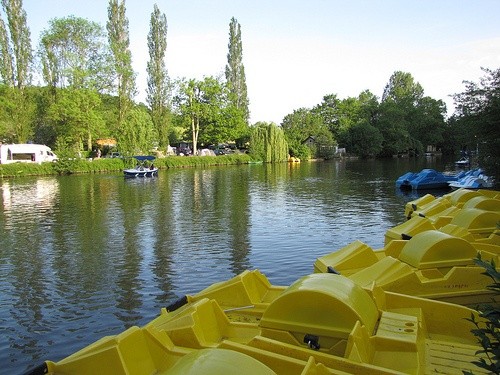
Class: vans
1,144,57,164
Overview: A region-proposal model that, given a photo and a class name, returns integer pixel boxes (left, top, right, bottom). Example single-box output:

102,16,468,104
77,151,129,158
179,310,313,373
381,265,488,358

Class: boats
15,155,500,375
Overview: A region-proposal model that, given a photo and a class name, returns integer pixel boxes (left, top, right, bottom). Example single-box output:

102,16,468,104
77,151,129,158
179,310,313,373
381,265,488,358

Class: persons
136,160,153,171
